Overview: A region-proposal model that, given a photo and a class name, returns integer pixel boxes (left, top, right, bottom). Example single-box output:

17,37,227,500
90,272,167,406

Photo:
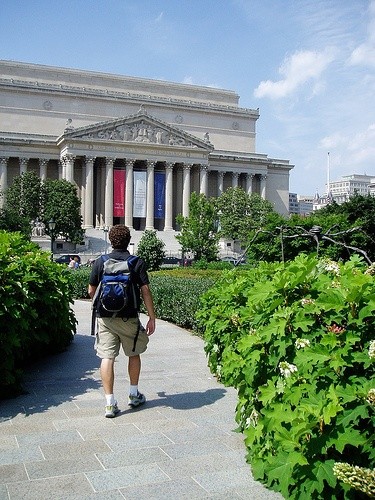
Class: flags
133,172,147,218
154,172,165,218
113,169,126,217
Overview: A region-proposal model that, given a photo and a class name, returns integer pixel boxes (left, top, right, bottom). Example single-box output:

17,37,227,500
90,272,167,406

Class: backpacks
91,255,140,352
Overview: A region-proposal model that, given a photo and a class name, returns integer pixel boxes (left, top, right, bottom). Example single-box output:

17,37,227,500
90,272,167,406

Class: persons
88,225,156,418
74,256,79,269
67,255,75,271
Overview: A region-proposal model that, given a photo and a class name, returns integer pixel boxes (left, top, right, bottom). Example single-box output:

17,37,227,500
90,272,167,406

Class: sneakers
128,389,146,408
105,400,121,418
